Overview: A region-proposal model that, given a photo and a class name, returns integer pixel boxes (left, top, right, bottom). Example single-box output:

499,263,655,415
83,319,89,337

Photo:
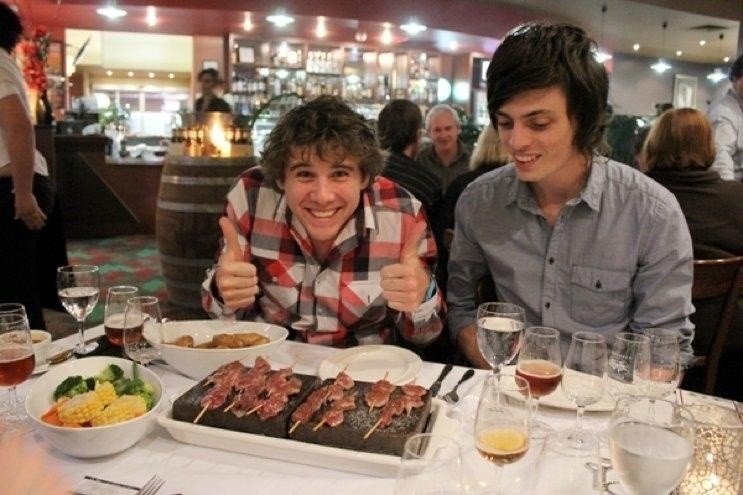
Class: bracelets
422,269,438,304
11,189,33,195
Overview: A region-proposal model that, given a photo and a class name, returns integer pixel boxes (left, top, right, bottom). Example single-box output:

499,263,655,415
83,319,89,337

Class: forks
131,474,166,495
441,368,475,403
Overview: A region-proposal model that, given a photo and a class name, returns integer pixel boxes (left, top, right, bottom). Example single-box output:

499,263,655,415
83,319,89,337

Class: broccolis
52,364,154,409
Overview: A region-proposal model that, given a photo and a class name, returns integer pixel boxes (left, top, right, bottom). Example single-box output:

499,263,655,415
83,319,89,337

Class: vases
32,93,51,125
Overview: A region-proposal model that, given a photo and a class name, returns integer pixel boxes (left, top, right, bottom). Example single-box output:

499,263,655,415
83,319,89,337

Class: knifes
430,363,454,397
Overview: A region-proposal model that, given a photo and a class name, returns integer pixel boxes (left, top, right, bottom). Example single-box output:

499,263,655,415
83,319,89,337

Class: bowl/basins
142,320,289,381
24,356,163,459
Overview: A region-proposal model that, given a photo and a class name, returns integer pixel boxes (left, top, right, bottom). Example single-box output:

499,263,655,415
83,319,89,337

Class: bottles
170,128,254,159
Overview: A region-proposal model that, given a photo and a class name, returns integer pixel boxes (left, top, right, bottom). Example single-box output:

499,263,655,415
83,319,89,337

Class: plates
318,344,424,387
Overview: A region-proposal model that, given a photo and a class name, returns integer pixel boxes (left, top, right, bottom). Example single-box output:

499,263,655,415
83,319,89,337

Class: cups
608,394,694,495
397,433,463,495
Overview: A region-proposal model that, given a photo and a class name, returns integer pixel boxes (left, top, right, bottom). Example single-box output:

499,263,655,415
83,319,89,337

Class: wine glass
515,325,564,439
559,332,609,450
471,374,532,493
475,303,527,399
0,264,164,424
633,328,682,425
599,333,651,445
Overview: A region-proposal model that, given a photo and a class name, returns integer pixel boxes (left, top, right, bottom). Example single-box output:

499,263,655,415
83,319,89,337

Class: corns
58,383,145,428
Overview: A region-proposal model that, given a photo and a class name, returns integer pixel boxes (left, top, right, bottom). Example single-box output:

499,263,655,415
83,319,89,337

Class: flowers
19,25,52,92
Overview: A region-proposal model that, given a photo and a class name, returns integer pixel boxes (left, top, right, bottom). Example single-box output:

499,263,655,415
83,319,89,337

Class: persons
703,52,743,185
412,99,476,196
0,0,77,334
440,21,697,393
636,107,742,370
194,67,233,113
197,91,448,361
362,98,442,237
437,118,515,233
601,99,673,171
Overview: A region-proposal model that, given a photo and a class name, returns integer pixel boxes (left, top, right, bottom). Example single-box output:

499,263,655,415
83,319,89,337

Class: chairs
685,255,742,394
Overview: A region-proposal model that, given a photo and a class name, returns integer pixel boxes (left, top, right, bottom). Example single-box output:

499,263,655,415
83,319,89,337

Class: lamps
263,4,295,27
704,31,729,84
649,21,671,74
94,1,126,20
593,4,612,63
398,13,427,35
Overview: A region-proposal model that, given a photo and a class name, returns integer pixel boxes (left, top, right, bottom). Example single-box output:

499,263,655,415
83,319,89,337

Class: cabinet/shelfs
306,44,443,119
468,55,493,124
223,32,308,126
